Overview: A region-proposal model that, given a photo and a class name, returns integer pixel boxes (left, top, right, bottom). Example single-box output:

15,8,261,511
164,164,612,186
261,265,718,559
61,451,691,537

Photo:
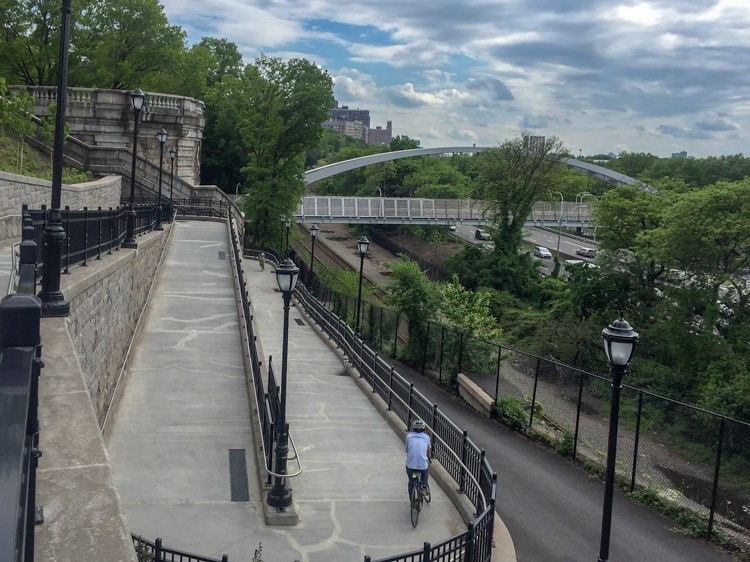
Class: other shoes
409,501,415,507
421,488,428,495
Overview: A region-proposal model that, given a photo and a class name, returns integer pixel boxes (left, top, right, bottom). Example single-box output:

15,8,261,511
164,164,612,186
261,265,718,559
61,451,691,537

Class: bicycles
409,454,431,528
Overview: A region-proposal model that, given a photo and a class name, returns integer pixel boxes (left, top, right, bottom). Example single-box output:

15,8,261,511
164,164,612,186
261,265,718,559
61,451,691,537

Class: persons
405,419,431,508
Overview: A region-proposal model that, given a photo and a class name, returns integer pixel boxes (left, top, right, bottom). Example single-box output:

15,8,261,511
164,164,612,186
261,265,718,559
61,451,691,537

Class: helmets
413,419,425,429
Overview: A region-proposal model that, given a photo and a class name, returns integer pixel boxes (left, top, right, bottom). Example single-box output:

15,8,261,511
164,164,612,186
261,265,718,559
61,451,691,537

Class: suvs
475,229,491,240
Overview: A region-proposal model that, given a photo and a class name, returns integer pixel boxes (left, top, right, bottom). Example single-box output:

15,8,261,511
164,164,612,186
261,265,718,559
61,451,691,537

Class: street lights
265,251,302,508
169,146,176,200
309,223,319,271
280,216,286,249
152,126,168,231
285,218,292,251
121,84,146,249
354,234,370,336
597,310,640,562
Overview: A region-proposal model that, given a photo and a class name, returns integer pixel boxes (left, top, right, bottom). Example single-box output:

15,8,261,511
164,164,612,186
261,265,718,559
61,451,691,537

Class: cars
575,248,596,258
535,247,552,259
448,226,456,231
